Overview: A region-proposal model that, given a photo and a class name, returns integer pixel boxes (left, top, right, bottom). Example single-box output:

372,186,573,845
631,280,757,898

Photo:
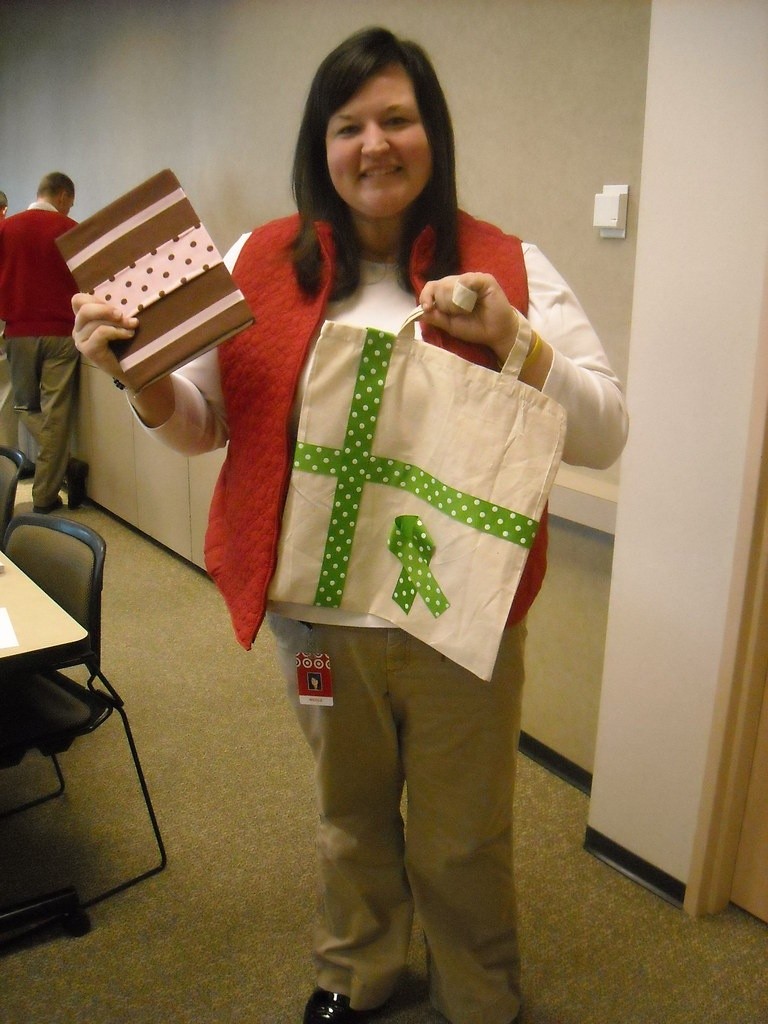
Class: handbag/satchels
266,278,567,683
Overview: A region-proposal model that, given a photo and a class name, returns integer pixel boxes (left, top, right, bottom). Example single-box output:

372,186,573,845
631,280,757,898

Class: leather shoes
303,986,351,1024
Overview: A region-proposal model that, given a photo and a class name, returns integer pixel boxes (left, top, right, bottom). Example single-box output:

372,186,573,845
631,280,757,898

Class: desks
0,547,92,936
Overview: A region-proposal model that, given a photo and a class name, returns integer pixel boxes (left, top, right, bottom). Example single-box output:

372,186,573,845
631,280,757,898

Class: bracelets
497,330,542,370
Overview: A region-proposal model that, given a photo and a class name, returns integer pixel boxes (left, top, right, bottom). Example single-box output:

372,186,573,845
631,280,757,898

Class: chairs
0,444,26,553
0,511,168,951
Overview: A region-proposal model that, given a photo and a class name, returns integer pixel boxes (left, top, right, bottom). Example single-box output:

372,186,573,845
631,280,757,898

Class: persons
0,191,35,480
0,172,89,514
71,27,629,1024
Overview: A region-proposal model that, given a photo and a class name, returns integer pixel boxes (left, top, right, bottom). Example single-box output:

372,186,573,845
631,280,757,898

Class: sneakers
33,495,63,514
66,457,89,510
